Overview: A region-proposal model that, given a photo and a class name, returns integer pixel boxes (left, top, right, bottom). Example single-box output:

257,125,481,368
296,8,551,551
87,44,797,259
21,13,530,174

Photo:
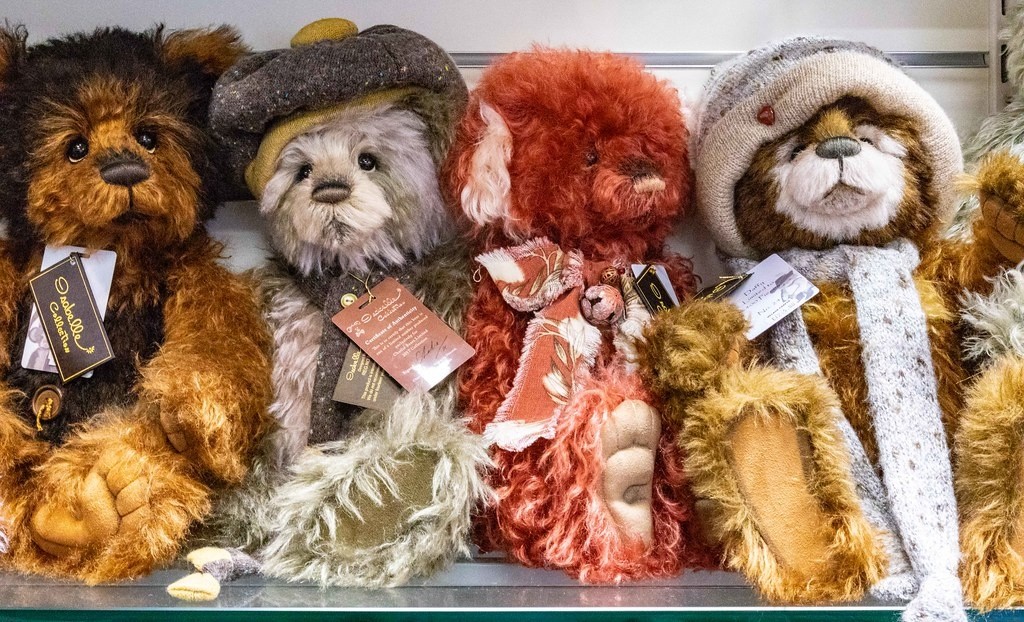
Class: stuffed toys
0,22,277,584
434,42,738,593
639,35,1023,621
177,16,473,594
